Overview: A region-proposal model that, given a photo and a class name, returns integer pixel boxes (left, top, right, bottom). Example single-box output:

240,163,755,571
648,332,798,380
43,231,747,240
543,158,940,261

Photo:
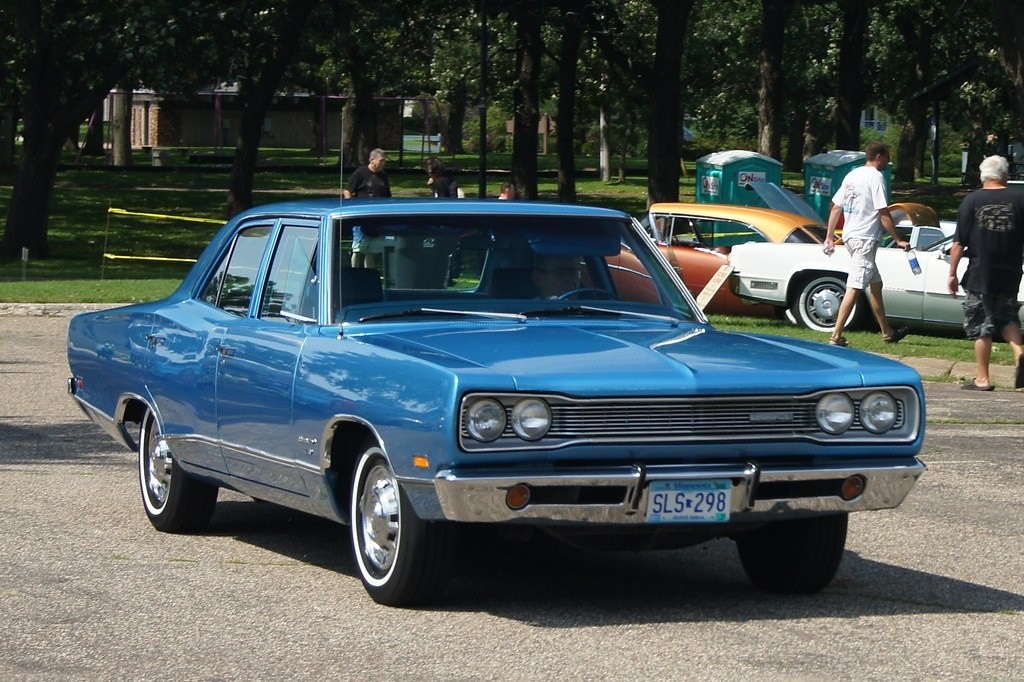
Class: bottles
907,250,922,275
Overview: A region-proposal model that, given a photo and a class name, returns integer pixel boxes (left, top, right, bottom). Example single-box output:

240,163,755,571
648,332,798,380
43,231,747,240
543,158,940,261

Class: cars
582,202,842,323
63,195,928,610
726,221,1024,338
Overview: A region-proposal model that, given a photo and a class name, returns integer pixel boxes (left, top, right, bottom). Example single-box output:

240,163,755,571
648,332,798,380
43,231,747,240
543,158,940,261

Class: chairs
331,266,386,323
488,267,541,300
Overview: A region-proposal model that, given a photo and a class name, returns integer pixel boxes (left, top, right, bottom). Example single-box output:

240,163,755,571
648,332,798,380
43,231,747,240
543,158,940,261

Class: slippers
961,378,994,391
1014,355,1024,387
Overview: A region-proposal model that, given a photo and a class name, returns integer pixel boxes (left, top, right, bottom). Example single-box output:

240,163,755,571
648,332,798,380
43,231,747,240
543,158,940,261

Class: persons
497,182,517,202
526,250,598,305
423,158,463,286
820,140,914,352
946,155,1024,391
341,148,392,267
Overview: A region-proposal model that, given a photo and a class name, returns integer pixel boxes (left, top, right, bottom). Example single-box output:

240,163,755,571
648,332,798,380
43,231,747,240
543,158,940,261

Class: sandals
830,337,850,348
883,326,910,344
